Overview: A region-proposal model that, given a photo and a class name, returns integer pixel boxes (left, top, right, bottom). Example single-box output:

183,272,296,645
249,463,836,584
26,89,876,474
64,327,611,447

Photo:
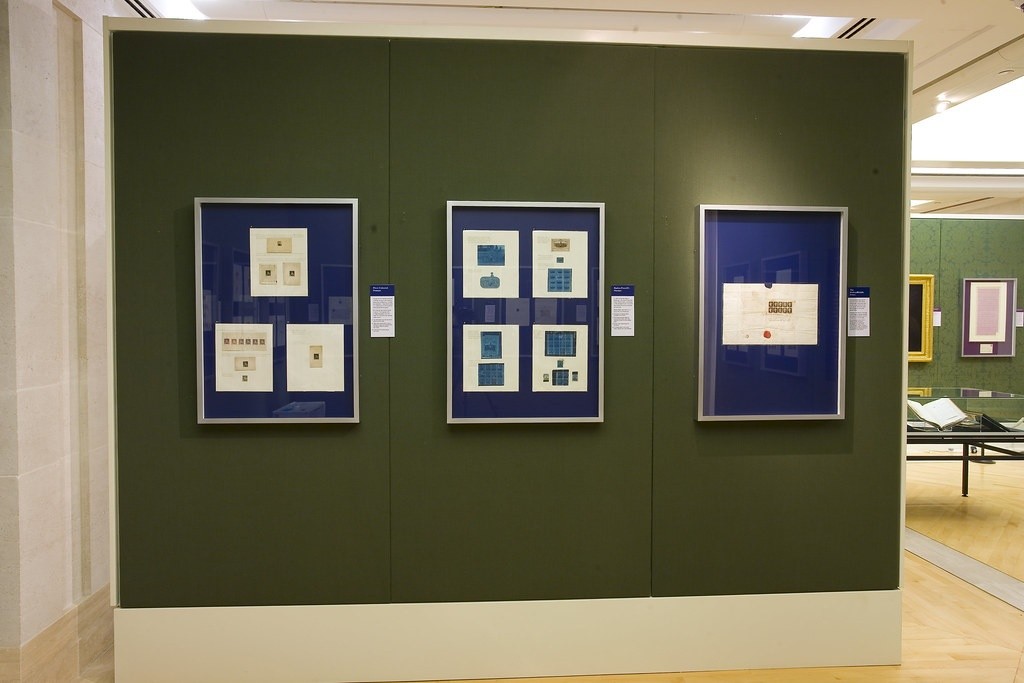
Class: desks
907,386,1024,497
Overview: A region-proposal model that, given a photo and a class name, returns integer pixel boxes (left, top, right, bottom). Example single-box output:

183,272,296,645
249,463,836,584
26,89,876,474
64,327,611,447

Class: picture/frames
908,388,932,397
907,274,935,363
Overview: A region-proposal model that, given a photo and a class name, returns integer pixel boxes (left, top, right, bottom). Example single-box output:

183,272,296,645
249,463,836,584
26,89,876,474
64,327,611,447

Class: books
906,398,968,432
